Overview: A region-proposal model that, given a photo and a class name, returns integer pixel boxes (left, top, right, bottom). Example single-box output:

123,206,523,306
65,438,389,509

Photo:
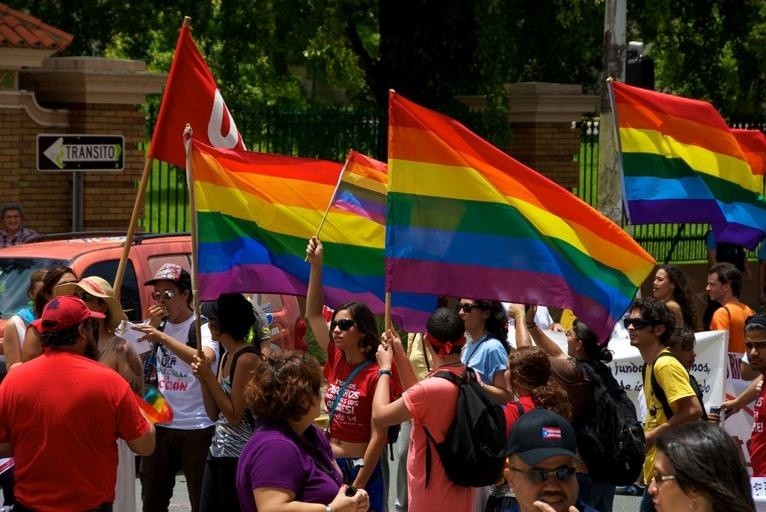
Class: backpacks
432,368,506,486
580,365,646,486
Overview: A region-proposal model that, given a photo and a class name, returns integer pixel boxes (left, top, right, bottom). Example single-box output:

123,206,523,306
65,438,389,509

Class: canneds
710,406,720,424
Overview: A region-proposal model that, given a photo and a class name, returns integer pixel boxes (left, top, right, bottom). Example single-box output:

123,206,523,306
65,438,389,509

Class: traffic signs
36,134,122,171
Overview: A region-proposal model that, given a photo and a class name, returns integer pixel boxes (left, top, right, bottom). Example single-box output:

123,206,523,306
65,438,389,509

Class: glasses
457,303,478,313
624,317,655,329
331,319,354,330
510,466,577,483
151,289,176,301
654,473,676,483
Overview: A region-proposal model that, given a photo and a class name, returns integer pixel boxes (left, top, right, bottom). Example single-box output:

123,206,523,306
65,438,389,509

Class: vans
0,231,309,365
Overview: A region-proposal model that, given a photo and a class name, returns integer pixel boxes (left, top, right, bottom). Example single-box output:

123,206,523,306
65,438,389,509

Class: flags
181,127,438,334
333,151,388,229
610,80,766,248
147,21,247,171
385,92,657,346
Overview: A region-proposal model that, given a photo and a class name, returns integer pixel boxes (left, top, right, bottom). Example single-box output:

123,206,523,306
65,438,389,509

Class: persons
1,202,765,512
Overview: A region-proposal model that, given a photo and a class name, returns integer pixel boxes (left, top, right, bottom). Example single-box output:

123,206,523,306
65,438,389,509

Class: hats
201,294,255,326
41,295,106,331
507,407,581,465
56,276,122,331
144,263,194,298
